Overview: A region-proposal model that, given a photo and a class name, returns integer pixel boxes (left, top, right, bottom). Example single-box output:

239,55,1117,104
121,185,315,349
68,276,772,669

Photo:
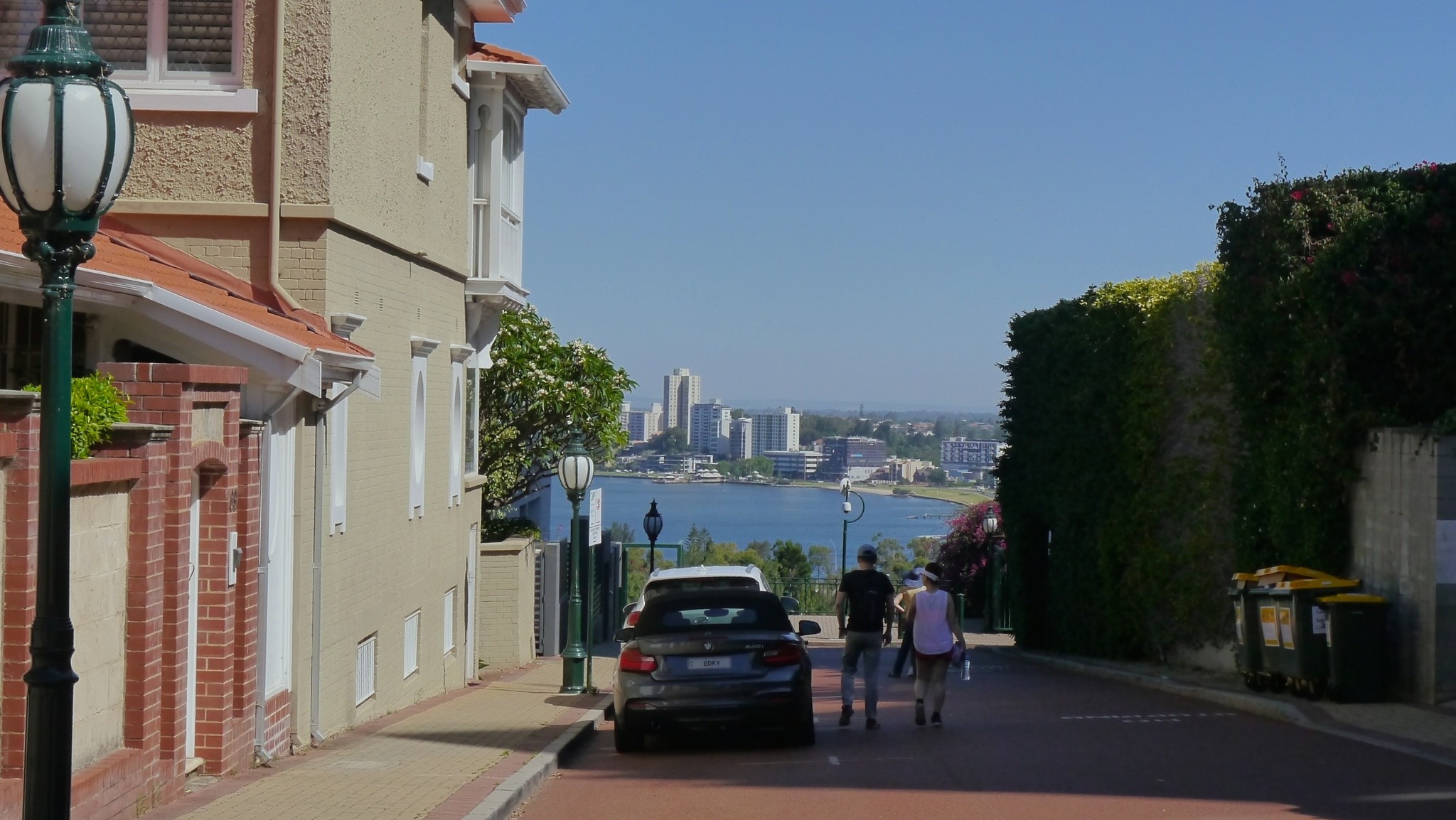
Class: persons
834,544,894,730
906,562,966,727
887,570,927,678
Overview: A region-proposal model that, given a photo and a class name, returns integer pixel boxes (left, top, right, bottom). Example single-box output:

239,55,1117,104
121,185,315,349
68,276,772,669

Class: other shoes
931,715,941,727
839,705,854,727
887,671,900,678
915,702,926,726
866,719,879,729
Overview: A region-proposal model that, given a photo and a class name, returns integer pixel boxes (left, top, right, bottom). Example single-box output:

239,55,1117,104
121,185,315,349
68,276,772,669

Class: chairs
732,609,769,629
659,610,693,631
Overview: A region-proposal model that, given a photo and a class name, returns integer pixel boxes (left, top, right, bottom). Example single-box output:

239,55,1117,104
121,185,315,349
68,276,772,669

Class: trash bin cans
1316,592,1391,703
1228,573,1263,688
1247,567,1362,700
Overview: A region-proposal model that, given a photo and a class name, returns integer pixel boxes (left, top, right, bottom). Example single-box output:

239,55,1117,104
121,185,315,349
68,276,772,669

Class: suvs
619,564,774,652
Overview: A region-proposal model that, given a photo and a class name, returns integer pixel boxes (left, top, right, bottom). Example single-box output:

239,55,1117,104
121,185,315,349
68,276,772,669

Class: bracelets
838,628,845,630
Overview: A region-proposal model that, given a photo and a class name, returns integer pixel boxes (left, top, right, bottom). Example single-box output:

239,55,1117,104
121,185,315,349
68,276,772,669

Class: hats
902,567,923,588
857,544,876,556
916,566,938,581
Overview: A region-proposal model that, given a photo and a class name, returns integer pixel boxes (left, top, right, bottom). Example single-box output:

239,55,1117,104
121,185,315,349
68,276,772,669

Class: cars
612,591,821,753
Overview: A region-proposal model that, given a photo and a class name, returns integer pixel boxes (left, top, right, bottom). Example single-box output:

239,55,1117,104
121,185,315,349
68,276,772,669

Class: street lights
838,475,865,576
0,0,136,820
643,499,664,572
557,426,594,692
982,505,998,630
